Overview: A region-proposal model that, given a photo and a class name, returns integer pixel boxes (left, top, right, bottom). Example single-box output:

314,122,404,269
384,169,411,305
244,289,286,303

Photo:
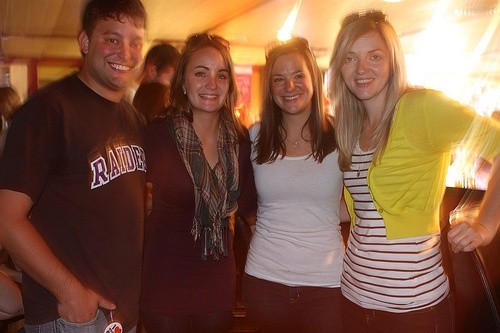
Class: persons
0,10,500,333
0,0,149,333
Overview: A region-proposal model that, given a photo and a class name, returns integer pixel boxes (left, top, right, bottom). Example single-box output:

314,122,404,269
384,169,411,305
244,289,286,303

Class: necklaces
353,144,370,178
281,131,311,149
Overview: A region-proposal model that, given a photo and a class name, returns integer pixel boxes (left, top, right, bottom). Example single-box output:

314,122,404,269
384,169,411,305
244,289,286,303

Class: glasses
185,33,230,50
341,10,387,27
265,37,309,63
